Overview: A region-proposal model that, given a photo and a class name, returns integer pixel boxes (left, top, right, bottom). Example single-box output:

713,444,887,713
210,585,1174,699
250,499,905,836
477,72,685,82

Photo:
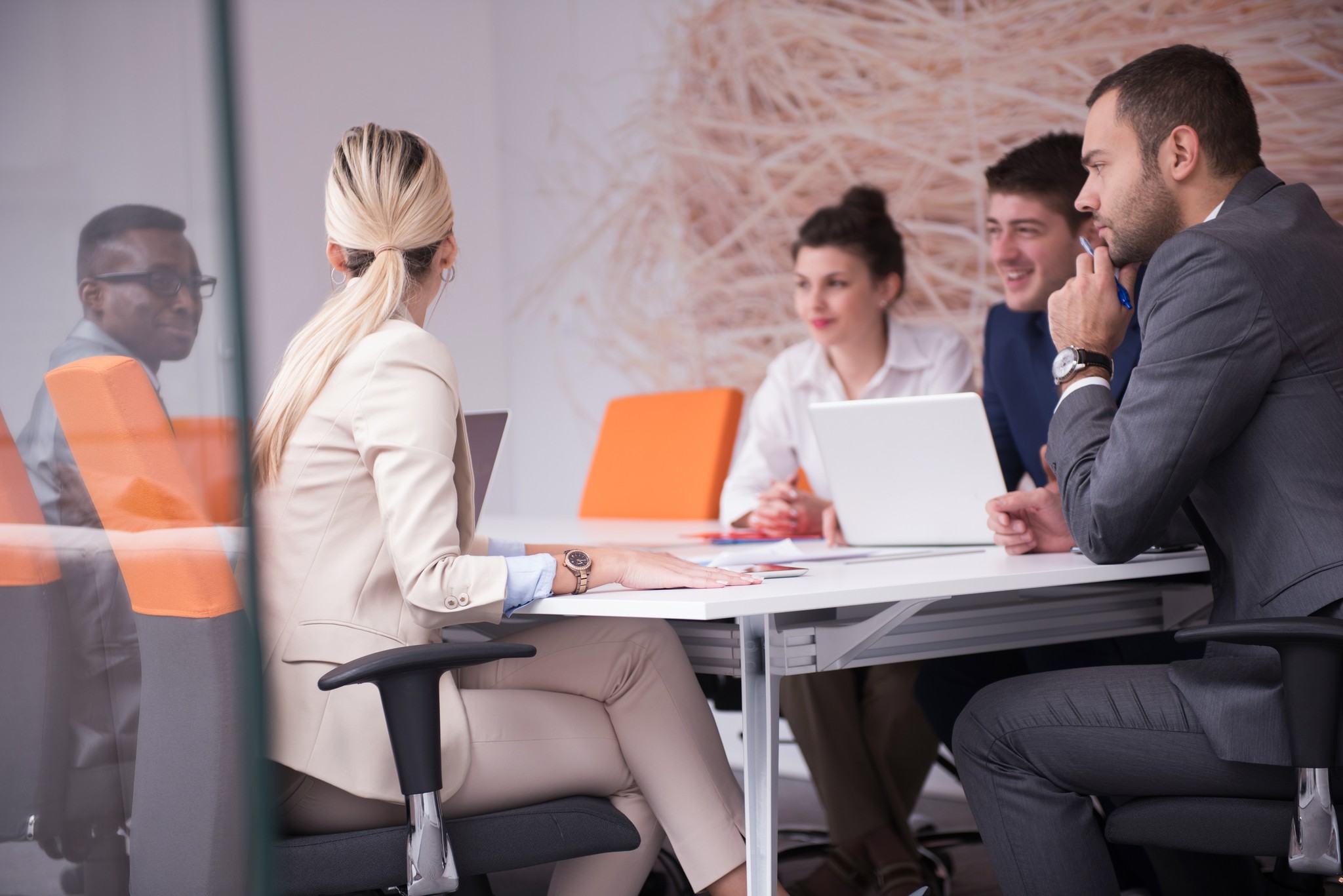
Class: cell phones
714,564,809,580
1070,542,1200,553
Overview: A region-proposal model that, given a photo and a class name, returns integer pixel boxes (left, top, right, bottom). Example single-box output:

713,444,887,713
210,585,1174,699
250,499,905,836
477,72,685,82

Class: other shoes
874,817,925,895
813,847,871,893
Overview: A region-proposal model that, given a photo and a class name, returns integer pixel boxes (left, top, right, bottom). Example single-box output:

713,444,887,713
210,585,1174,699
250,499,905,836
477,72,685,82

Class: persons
15,206,221,730
952,42,1342,896
720,180,976,896
246,120,795,896
818,134,1204,769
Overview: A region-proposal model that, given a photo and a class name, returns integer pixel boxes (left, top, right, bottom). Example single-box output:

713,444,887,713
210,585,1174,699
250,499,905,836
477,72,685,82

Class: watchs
559,546,593,594
1053,345,1115,390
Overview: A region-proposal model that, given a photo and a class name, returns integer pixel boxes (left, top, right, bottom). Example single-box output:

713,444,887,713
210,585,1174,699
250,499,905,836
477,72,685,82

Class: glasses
94,266,218,299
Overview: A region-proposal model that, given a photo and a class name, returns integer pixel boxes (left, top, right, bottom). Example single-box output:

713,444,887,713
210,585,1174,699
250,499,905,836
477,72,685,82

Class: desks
439,531,1216,896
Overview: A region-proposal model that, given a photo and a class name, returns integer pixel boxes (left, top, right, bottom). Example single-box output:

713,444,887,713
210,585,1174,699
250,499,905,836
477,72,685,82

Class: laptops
808,393,1010,547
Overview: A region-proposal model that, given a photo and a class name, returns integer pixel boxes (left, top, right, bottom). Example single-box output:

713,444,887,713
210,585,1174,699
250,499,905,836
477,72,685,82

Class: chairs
575,387,743,526
3,412,141,896
45,354,646,896
